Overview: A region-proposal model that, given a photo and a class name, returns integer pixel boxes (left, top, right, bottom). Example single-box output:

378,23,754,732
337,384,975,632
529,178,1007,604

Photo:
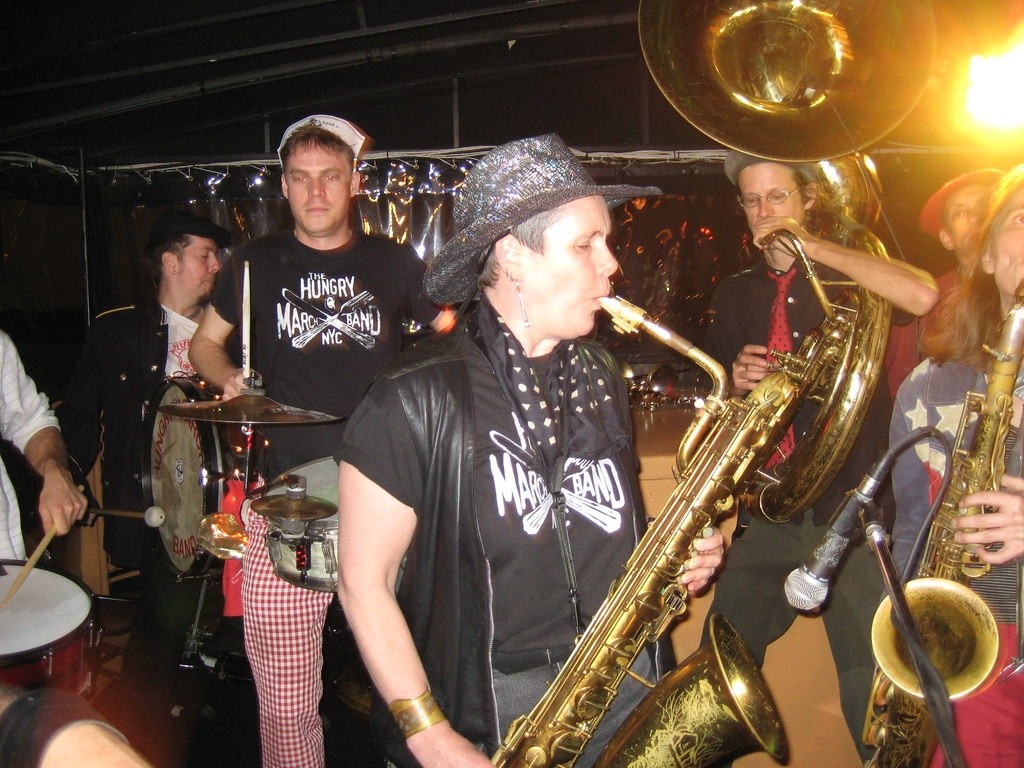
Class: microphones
784,453,896,610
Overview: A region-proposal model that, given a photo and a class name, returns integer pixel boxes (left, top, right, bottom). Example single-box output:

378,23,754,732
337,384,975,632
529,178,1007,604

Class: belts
492,644,578,675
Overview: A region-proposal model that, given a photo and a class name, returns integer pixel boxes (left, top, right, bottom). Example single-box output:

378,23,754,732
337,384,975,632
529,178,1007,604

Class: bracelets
388,688,449,739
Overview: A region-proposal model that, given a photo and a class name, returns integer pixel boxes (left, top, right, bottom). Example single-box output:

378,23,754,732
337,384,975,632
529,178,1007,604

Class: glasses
736,185,802,209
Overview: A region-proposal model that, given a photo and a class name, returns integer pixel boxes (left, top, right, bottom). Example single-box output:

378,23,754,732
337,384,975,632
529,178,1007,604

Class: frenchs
635,0,941,526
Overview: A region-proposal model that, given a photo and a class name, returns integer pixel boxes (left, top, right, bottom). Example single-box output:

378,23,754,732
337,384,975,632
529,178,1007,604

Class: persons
0,324,88,562
699,153,911,768
192,117,465,768
887,160,1024,768
58,210,234,724
338,135,727,768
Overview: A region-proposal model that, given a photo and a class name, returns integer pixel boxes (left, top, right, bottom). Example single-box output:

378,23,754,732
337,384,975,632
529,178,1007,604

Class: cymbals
250,493,338,521
156,394,348,426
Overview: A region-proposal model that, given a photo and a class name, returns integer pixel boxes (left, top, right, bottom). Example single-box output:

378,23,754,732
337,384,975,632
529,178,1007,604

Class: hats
275,114,365,173
150,209,233,261
421,133,664,307
920,164,1004,242
724,148,820,187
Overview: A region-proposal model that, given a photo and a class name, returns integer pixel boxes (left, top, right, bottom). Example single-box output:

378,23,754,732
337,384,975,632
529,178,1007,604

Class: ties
764,268,797,472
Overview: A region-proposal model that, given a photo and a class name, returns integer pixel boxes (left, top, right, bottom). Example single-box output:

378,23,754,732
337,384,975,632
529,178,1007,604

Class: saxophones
861,280,1024,767
489,293,855,768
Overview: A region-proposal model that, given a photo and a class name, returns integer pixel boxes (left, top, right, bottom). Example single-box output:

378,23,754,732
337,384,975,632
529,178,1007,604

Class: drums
267,456,339,592
144,376,269,580
0,558,100,701
